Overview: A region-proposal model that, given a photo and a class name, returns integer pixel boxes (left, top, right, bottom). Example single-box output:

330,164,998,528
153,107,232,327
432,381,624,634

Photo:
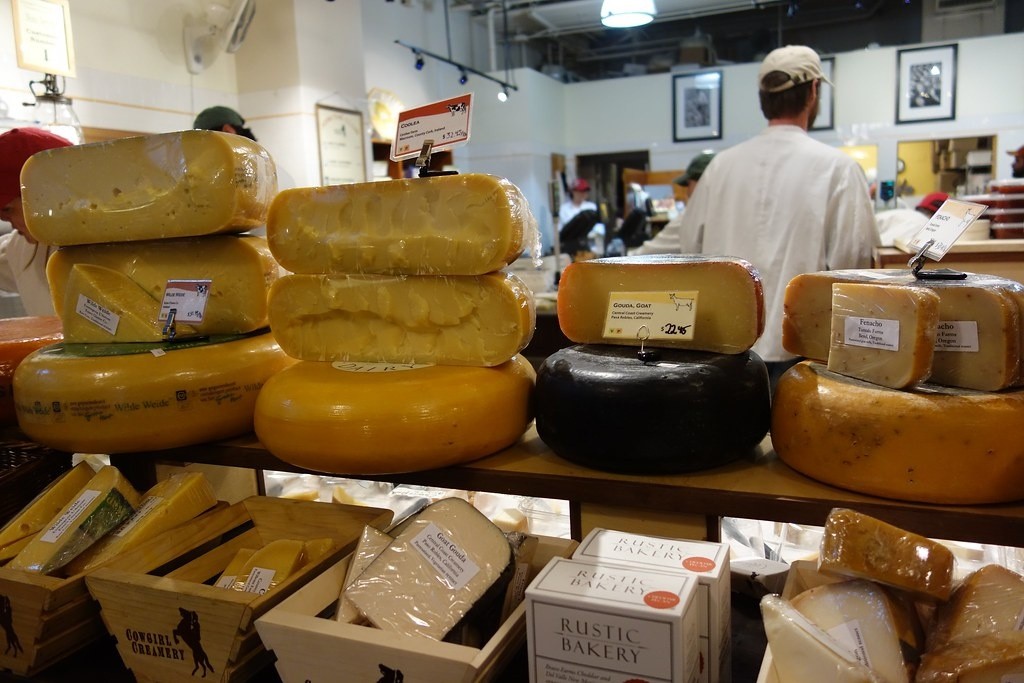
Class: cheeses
0,129,536,476
555,252,766,355
760,507,1024,683
0,460,540,643
772,266,1024,503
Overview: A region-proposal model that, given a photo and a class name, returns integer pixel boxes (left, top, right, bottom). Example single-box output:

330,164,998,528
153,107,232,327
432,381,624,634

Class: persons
193,106,297,277
559,178,604,248
680,45,882,382
630,150,717,254
1006,146,1024,178
0,127,73,316
877,192,950,245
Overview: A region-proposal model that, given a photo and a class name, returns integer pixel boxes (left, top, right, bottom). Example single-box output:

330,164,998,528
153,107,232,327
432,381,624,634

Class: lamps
599,0,659,28
497,83,510,103
459,67,470,85
411,48,424,71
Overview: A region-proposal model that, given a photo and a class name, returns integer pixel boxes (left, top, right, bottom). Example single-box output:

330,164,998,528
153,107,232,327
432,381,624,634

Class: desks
869,237,1024,284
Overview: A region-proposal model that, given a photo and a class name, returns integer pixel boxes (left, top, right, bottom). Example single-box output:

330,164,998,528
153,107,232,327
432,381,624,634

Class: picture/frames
807,58,836,132
895,43,959,123
672,70,724,143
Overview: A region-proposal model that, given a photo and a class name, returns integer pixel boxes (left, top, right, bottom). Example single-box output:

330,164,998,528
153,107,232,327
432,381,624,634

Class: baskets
1,436,73,528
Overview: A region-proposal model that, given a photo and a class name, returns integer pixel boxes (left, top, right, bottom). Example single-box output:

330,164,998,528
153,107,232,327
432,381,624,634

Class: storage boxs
948,151,965,169
938,172,961,195
968,174,992,196
525,555,703,683
256,530,575,683
0,499,229,677
949,138,980,152
964,149,992,167
938,150,948,170
573,526,731,683
88,491,396,683
757,557,851,683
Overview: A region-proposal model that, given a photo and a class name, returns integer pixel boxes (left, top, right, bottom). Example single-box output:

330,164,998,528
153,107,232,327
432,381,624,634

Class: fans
183,0,257,75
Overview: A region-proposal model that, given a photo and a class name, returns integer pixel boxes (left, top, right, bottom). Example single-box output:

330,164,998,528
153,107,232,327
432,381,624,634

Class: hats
0,128,76,210
916,191,950,214
572,178,590,191
194,106,246,132
675,151,717,186
757,45,834,93
1008,146,1024,156
